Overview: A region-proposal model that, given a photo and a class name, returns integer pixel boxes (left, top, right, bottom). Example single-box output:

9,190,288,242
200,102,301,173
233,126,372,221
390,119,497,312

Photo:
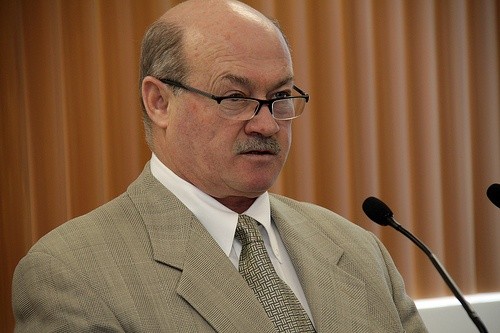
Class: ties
235,214,318,333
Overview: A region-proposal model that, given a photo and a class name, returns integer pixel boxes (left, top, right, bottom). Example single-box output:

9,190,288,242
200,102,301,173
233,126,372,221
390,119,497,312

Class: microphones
361,197,489,332
487,183,500,207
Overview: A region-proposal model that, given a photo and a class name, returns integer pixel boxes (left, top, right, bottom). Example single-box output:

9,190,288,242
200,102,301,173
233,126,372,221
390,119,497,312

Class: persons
12,0,429,333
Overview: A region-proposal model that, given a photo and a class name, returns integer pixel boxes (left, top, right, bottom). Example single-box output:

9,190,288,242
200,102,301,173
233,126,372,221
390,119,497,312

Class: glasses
151,76,309,120
